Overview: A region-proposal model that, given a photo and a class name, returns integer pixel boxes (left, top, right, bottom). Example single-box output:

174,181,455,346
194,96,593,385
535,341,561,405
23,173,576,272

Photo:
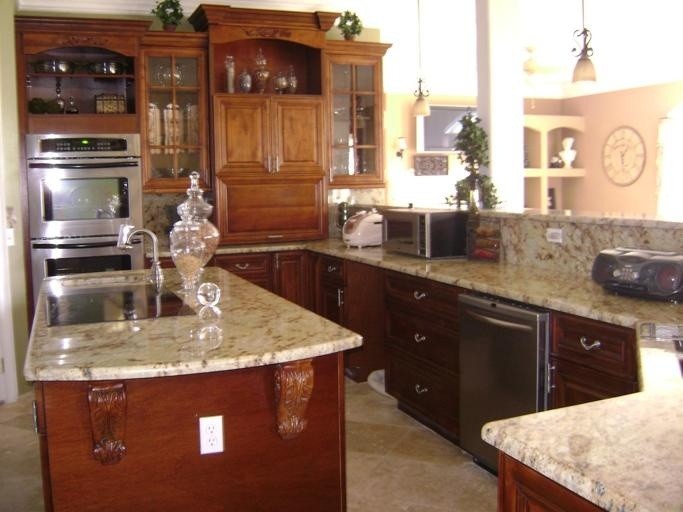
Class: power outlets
199,415,225,455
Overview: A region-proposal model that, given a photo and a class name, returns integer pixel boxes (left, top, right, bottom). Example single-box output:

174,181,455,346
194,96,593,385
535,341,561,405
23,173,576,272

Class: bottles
53,86,65,113
223,54,235,93
65,96,79,113
339,201,348,225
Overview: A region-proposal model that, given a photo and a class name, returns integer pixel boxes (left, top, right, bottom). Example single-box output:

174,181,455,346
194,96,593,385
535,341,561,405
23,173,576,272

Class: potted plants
338,11,363,40
152,0,184,32
445,108,499,208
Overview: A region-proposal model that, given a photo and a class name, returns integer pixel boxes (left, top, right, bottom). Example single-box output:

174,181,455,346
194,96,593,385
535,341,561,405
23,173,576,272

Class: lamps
571,0,597,81
411,1,431,117
396,136,407,157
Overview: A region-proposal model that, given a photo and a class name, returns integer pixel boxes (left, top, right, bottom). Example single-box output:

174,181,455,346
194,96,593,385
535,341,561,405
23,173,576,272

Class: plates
156,62,184,87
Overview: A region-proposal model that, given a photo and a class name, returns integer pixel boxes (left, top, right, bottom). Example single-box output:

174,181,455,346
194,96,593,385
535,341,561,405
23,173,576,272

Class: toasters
342,213,382,247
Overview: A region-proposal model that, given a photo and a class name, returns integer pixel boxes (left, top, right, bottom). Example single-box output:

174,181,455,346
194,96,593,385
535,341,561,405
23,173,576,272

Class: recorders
592,248,683,303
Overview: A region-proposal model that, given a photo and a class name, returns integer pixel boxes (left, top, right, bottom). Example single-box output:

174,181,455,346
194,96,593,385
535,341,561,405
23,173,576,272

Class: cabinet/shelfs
140,30,210,193
190,3,341,243
524,112,585,210
383,270,459,440
271,251,314,310
546,310,636,411
320,255,384,383
217,254,271,289
325,40,392,188
14,14,153,134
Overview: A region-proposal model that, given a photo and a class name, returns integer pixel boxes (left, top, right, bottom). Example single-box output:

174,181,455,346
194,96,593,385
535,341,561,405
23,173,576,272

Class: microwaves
381,208,467,260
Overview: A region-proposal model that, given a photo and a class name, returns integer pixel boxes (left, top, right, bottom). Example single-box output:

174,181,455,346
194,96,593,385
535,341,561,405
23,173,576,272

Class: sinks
60,275,139,285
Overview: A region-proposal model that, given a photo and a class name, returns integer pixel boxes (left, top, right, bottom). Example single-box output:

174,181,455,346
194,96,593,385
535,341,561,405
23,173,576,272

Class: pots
86,59,123,75
26,59,76,74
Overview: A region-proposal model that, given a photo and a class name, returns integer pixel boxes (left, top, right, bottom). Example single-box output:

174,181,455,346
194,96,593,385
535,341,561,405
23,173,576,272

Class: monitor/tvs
416,104,476,154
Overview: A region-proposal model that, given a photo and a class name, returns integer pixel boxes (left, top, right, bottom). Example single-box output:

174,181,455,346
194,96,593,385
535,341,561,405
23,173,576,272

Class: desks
441,202,502,209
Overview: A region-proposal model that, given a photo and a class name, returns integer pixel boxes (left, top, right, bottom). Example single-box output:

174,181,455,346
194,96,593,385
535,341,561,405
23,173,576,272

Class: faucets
126,227,164,277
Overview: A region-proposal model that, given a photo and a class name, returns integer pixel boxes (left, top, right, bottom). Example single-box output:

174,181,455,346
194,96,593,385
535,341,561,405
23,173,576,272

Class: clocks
602,127,646,187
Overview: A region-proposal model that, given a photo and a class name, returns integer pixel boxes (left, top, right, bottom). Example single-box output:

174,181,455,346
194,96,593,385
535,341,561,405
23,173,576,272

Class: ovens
26,158,146,311
40,286,148,327
